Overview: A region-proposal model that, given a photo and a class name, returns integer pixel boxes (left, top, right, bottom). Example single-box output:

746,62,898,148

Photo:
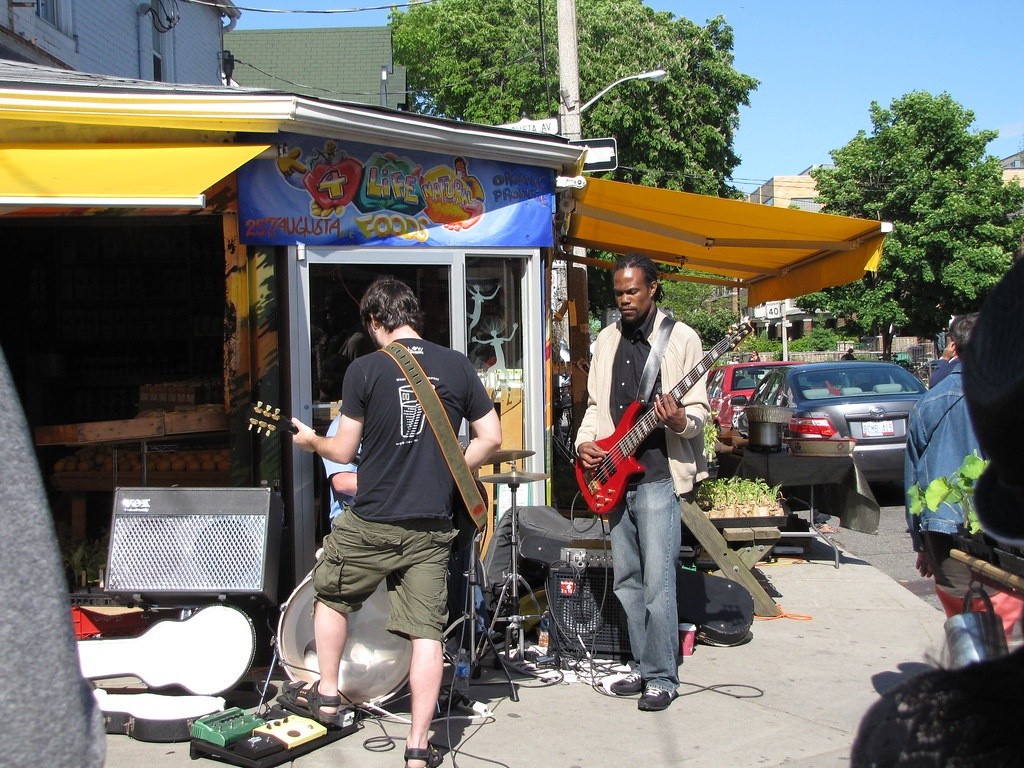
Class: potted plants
697,476,791,528
907,450,993,566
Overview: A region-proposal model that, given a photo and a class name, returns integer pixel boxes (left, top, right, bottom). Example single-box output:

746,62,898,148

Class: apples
53,445,231,473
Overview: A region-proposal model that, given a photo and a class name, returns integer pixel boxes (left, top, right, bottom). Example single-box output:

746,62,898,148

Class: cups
678,623,696,656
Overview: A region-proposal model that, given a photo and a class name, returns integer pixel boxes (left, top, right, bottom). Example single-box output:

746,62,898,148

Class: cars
704,360,841,434
730,360,930,483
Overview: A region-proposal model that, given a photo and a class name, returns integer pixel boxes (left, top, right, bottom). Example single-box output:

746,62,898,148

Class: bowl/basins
744,407,792,423
748,422,778,447
782,438,857,456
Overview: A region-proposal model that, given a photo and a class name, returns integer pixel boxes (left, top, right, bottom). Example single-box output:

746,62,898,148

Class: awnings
0,137,275,209
570,173,892,308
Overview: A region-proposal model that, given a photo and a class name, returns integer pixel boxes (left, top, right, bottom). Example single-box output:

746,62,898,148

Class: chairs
802,384,903,399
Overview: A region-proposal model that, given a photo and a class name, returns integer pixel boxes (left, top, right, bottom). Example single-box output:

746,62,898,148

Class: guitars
247,401,488,551
576,322,752,515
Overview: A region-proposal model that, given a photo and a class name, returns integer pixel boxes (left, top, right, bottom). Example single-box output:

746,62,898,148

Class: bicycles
876,352,938,388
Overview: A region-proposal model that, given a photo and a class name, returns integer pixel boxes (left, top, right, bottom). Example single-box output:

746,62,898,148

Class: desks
737,443,881,568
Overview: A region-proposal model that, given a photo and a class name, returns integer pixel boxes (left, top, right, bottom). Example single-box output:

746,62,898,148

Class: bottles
451,649,469,699
195,375,224,404
477,369,522,389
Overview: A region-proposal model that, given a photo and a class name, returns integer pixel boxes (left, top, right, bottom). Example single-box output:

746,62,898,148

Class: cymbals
474,471,552,483
480,450,536,465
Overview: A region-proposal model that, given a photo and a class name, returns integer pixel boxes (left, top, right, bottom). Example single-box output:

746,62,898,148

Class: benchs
711,527,779,616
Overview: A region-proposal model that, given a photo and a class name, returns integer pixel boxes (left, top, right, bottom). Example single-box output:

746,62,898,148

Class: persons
844,349,855,360
282,280,502,768
852,252,1024,767
729,356,739,364
929,335,960,389
320,414,507,650
573,254,711,712
1,344,107,768
322,305,376,360
748,351,760,362
899,313,1024,645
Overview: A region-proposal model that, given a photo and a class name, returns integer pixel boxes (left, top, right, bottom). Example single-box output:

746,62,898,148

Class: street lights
557,70,668,442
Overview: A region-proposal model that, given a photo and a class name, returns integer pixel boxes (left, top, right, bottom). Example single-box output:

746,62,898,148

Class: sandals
281,679,342,724
404,745,443,768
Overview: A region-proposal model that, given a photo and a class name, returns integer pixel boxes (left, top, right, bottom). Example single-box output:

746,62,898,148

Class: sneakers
638,682,675,711
466,629,511,656
610,668,644,695
